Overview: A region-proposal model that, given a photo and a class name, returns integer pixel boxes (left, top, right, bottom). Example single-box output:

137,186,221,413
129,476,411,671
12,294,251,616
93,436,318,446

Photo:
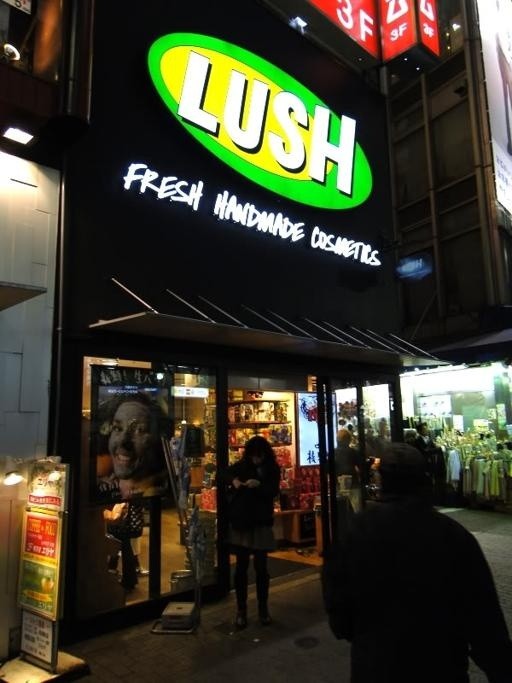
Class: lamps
4,455,62,486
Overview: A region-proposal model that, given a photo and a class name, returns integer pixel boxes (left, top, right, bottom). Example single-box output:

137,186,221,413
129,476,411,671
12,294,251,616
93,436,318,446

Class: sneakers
110,568,149,591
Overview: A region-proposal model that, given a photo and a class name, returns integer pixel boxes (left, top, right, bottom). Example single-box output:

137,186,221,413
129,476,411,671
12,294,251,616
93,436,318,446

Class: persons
108,394,175,496
222,436,280,633
320,440,511,682
326,421,440,512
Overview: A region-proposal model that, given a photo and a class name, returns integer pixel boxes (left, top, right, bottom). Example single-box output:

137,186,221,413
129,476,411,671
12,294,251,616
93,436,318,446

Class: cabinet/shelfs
201,397,314,546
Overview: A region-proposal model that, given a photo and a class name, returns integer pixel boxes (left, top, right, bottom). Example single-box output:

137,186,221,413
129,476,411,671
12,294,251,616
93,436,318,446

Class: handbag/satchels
106,502,143,538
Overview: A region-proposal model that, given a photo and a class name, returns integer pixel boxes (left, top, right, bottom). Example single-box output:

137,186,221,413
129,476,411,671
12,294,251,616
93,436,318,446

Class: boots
235,573,247,627
256,573,271,625
